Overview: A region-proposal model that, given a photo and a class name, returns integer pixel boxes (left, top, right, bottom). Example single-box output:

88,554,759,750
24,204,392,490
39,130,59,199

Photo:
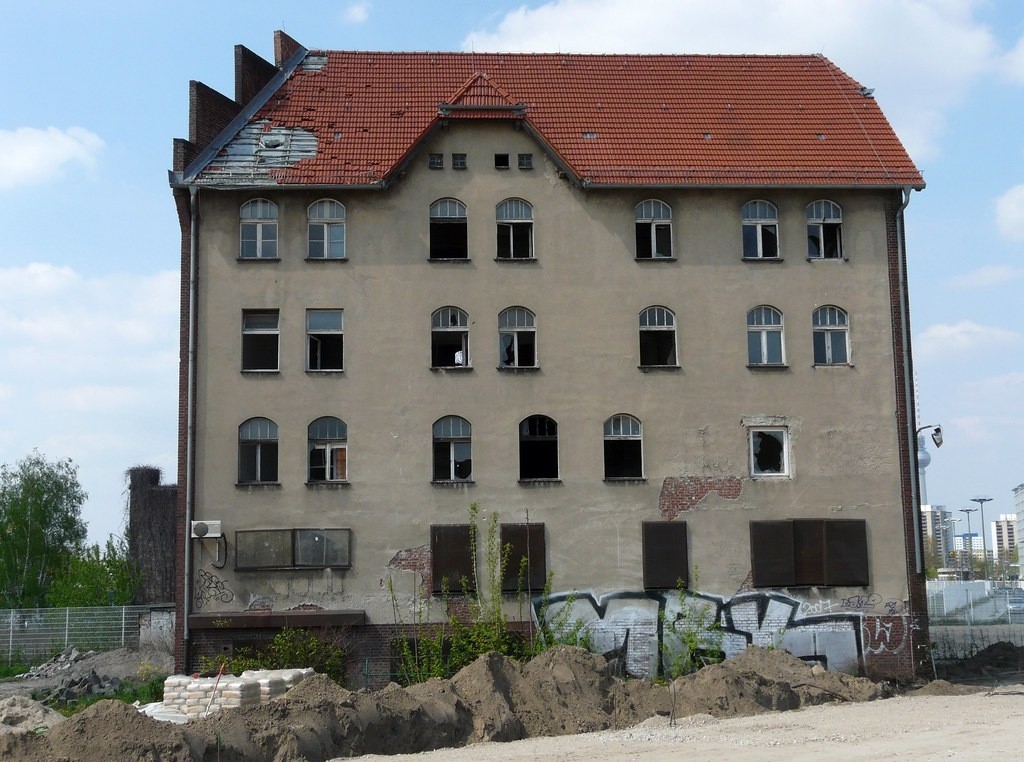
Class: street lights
970,496,994,580
935,525,950,568
944,517,962,582
958,508,978,581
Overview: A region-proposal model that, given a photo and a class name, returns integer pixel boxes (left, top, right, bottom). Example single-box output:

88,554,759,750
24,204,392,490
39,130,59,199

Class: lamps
916,424,944,448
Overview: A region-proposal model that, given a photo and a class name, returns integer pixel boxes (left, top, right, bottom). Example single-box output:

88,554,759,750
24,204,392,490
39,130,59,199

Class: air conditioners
191,520,221,538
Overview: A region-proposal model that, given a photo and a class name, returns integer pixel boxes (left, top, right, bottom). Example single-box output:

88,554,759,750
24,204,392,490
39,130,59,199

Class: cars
998,572,1019,582
993,586,1024,597
1006,597,1024,615
